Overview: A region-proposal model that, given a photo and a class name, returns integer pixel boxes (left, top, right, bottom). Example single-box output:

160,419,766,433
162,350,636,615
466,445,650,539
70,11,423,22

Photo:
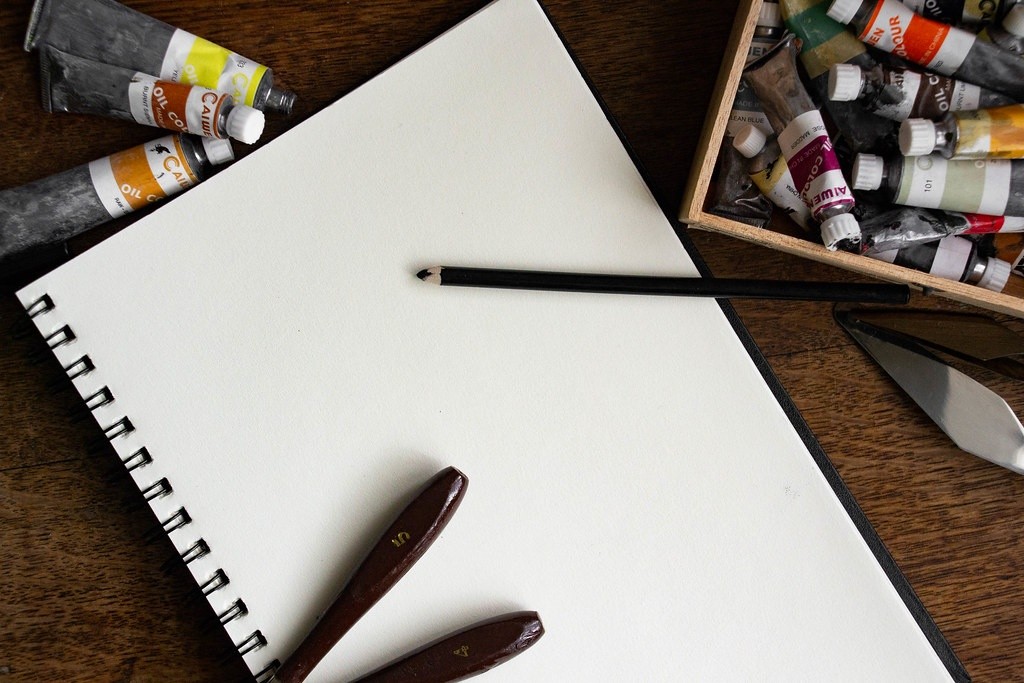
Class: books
9,0,969,682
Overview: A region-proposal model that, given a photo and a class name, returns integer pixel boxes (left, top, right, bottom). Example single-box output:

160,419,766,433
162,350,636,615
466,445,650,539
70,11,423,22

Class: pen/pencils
415,267,913,306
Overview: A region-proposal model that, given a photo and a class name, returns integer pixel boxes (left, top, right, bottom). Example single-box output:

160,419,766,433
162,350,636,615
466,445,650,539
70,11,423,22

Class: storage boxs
679,0,1024,321
